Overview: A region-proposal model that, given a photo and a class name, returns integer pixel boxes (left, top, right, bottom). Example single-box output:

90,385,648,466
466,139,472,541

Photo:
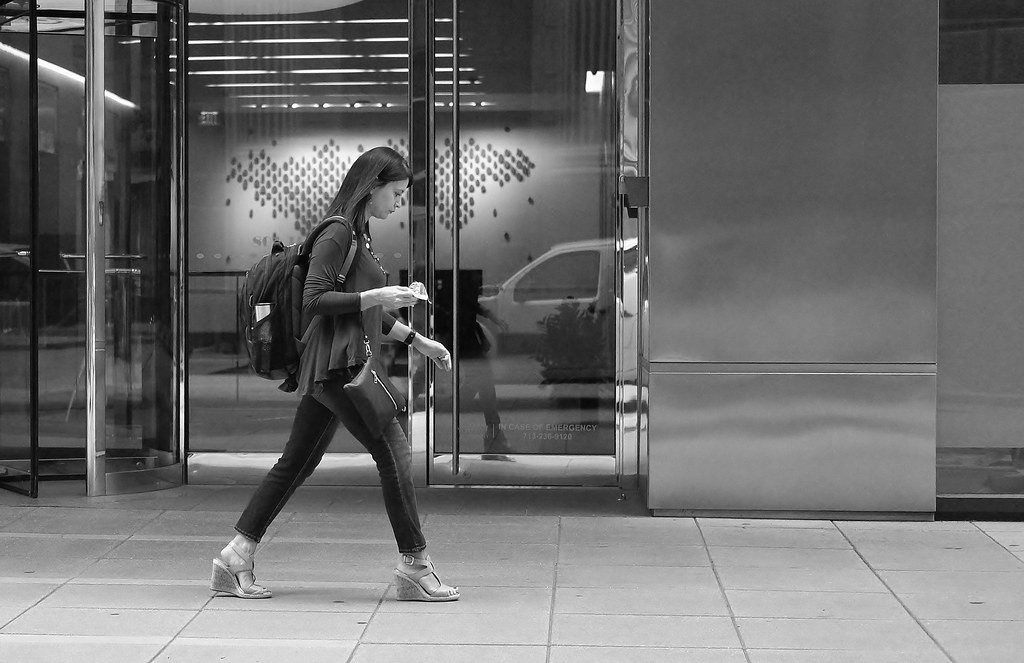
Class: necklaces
366,238,386,274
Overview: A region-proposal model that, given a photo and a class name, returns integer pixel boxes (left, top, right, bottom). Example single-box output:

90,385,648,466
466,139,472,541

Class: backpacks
237,215,357,394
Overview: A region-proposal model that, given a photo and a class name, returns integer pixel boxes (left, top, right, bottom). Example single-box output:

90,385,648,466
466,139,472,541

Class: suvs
416,239,647,408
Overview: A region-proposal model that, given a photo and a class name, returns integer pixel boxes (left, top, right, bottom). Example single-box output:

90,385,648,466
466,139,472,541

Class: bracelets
404,329,416,345
484,309,490,318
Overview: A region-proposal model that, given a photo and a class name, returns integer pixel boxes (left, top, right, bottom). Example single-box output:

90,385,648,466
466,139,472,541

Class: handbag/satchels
340,315,407,439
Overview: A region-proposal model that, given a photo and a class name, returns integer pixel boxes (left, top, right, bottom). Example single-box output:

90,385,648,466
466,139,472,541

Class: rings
439,357,441,358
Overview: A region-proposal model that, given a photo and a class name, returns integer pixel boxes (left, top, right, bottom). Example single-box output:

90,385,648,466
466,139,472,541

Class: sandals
395,551,460,603
484,427,522,454
211,539,274,599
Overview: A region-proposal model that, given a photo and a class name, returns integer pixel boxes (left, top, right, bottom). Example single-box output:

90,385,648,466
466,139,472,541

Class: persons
400,267,518,454
211,145,463,600
